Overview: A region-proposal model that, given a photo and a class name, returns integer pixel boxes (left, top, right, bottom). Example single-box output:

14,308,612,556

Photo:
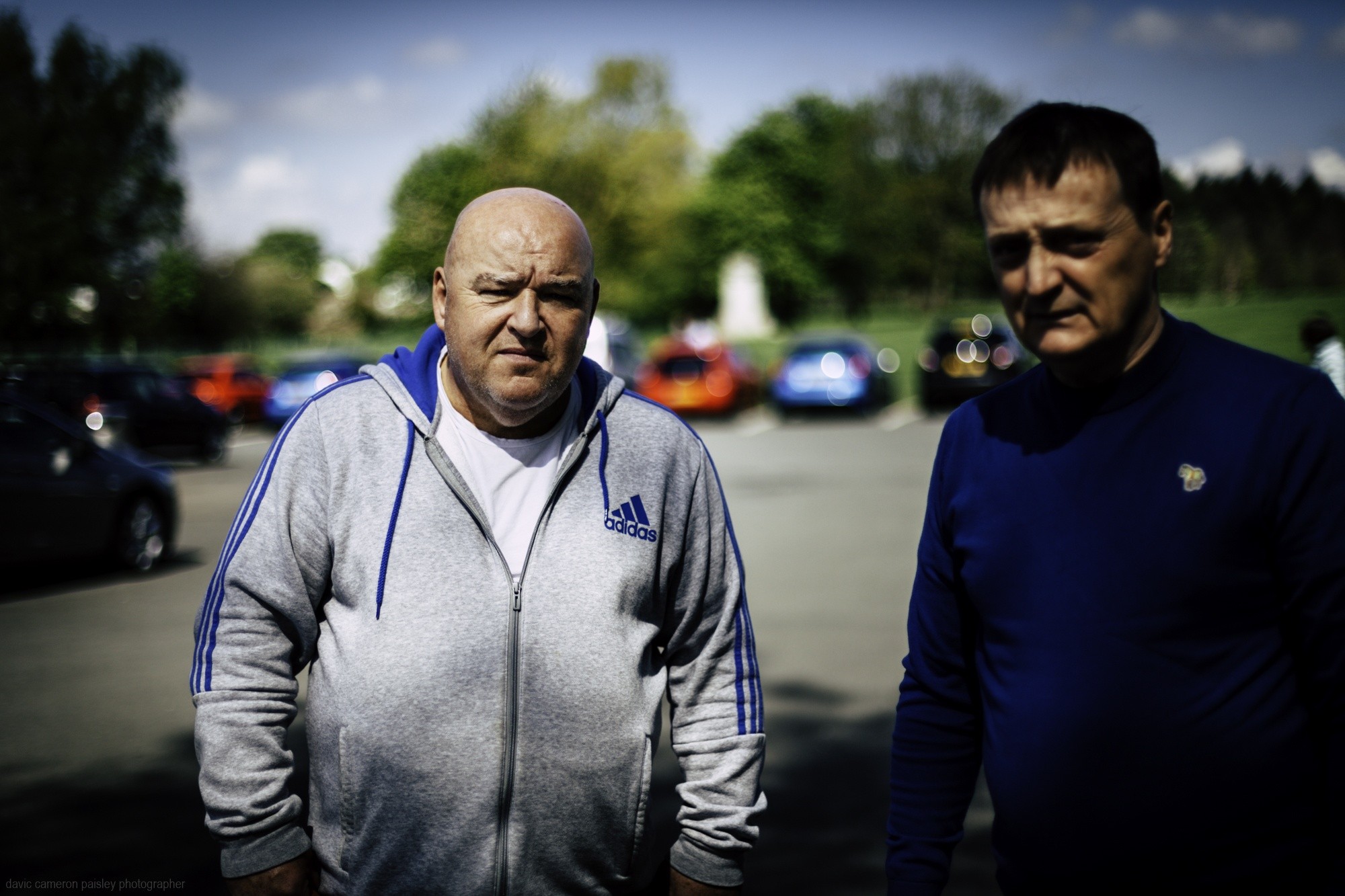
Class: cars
150,356,275,430
918,319,1026,416
767,336,890,415
0,383,186,590
262,353,384,427
0,355,234,468
634,332,753,419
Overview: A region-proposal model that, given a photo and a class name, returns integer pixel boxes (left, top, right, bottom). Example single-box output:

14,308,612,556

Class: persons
871,101,1344,896
191,186,766,896
